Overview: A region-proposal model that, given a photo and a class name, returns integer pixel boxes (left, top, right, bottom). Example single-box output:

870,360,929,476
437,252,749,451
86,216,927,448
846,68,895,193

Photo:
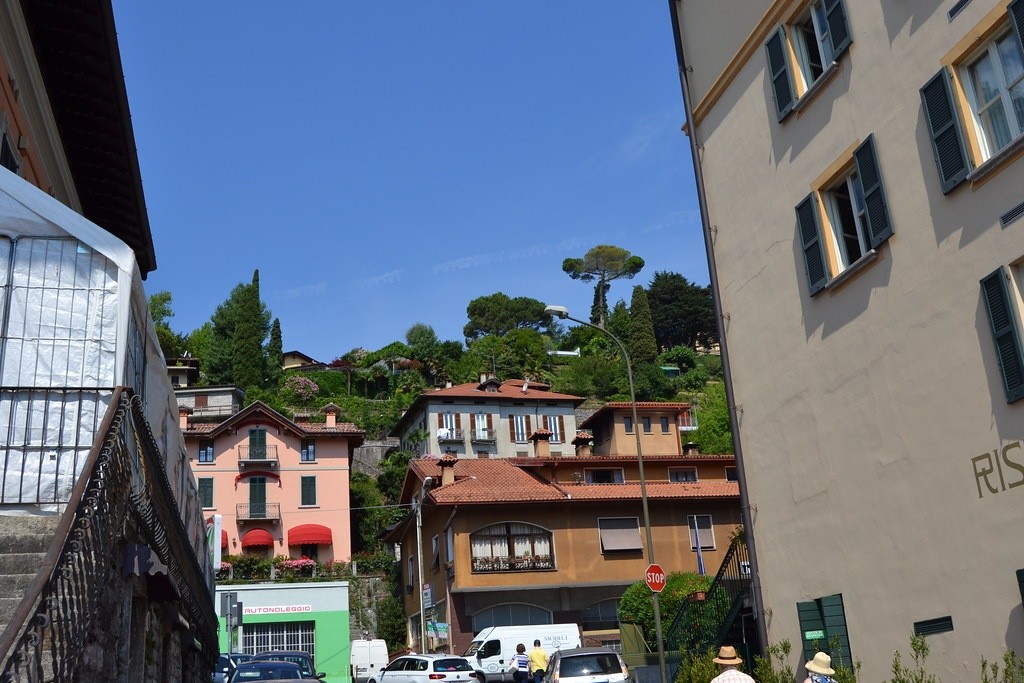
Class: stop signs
645,563,667,593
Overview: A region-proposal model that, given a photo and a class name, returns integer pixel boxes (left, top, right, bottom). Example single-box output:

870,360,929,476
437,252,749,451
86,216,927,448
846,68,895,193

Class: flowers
275,559,317,571
219,562,233,573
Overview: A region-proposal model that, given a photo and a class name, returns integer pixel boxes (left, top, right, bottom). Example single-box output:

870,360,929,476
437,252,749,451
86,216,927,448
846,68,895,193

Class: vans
349,638,390,683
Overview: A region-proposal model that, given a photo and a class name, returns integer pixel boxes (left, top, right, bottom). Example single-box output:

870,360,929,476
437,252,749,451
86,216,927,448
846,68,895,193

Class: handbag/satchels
508,654,519,674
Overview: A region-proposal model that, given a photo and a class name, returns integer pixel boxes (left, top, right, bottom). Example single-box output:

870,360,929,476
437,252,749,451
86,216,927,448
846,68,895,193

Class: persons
710,646,756,683
528,639,549,683
509,643,531,683
803,652,840,683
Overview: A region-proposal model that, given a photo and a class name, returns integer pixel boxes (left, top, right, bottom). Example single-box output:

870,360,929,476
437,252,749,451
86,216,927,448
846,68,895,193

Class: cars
540,646,634,683
224,660,321,683
249,650,326,679
211,652,253,683
365,653,482,683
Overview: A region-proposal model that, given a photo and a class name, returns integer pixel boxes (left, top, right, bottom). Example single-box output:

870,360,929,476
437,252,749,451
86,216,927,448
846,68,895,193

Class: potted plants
686,585,706,604
472,551,553,564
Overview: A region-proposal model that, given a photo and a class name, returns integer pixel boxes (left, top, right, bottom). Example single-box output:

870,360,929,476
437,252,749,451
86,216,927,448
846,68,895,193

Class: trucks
459,622,582,683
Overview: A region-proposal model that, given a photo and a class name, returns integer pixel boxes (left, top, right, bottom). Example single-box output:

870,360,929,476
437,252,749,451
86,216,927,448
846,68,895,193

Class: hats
805,652,835,676
712,646,743,664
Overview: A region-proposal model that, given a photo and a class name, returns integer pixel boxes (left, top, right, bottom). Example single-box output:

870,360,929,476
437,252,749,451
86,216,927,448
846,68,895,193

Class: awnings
221,530,227,547
243,529,273,549
288,524,332,546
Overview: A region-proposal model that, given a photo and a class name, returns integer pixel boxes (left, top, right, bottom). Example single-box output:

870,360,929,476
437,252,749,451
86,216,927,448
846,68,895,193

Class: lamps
278,536,283,544
233,538,236,545
256,422,259,428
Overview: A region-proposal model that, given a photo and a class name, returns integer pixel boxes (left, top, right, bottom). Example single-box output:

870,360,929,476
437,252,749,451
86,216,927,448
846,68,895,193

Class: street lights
414,475,433,654
544,304,670,683
